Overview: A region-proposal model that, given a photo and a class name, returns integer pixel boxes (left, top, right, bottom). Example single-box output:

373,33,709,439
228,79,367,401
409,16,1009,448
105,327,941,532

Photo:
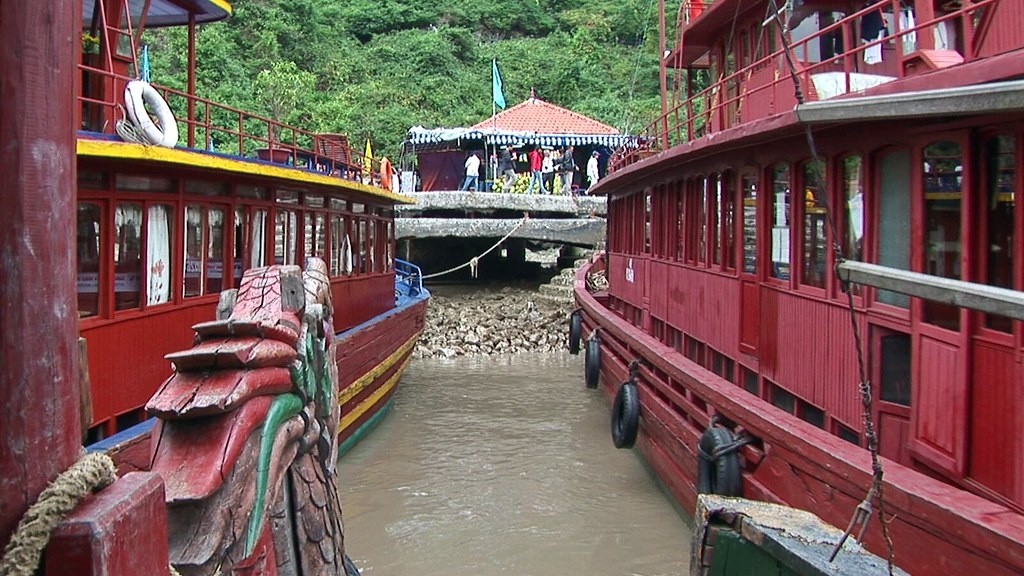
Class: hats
592,151,601,156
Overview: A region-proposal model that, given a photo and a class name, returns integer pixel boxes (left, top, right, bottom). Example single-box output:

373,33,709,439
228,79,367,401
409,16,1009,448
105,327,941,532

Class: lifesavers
569,312,581,355
697,427,745,499
124,80,179,149
379,157,392,190
584,339,600,390
611,382,640,450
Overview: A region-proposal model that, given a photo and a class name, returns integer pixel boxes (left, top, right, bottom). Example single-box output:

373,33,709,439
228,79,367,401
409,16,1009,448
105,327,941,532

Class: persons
528,145,551,195
502,144,517,193
554,145,579,197
539,150,556,195
461,150,481,191
584,151,601,197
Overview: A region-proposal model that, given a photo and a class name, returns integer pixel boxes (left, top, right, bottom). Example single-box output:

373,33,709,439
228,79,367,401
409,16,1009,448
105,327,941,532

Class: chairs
78,262,238,319
317,133,362,181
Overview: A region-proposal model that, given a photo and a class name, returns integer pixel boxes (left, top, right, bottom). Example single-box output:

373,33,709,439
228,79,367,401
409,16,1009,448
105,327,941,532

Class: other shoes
542,191,551,195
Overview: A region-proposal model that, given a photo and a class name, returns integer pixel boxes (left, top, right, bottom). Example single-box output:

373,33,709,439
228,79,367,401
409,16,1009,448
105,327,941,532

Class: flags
364,139,372,172
493,59,506,110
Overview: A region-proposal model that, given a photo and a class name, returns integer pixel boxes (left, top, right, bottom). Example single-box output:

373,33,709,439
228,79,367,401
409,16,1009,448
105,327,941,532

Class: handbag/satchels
558,163,568,173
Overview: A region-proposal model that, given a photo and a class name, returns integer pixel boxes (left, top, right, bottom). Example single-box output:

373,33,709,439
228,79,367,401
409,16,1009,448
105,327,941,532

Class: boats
565,1,1023,576
0,0,434,468
